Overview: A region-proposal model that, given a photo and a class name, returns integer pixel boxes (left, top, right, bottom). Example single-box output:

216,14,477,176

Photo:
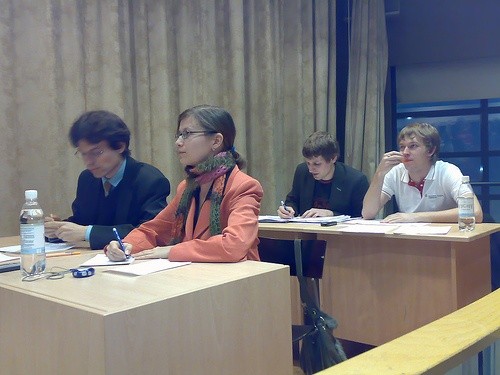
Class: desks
257,213,500,347
0,234,295,375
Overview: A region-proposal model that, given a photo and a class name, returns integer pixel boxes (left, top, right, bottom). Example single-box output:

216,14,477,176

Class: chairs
258,238,328,369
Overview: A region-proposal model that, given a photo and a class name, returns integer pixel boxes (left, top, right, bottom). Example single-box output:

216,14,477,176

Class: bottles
19,190,46,277
458,176,475,231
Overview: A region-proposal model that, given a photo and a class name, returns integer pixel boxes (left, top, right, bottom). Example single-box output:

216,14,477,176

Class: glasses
75,149,104,158
175,129,218,139
21,271,64,282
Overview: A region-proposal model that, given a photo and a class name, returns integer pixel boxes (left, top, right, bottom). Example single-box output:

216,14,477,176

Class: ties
104,182,110,197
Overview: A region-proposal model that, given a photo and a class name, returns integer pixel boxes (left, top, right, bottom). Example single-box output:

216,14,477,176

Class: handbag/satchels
301,304,348,375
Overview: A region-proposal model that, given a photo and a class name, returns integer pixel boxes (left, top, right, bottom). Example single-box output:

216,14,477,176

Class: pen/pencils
50,213,55,221
281,201,293,220
112,228,127,262
45,252,81,257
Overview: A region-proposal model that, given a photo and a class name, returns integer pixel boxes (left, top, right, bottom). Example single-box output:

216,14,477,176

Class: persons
276,131,370,220
103,104,264,263
361,122,483,224
44,110,170,250
